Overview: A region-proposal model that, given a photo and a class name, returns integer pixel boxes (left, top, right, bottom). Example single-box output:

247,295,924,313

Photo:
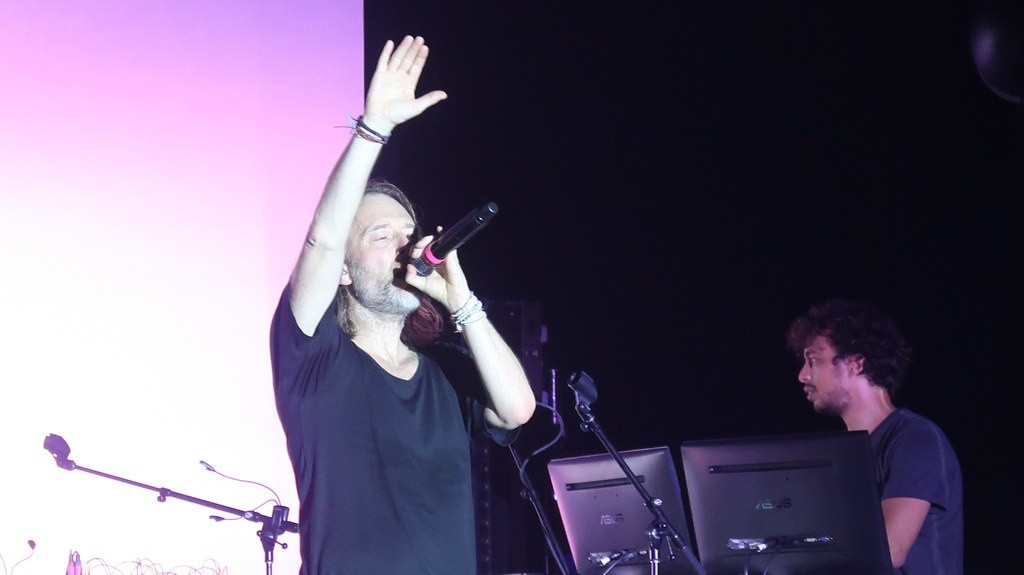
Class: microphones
407,202,498,277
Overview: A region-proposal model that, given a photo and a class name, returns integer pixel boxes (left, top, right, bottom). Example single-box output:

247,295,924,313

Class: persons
271,34,537,575
787,296,964,575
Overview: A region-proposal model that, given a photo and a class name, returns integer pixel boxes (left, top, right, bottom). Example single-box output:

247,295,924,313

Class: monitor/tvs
681,429,893,575
547,446,695,575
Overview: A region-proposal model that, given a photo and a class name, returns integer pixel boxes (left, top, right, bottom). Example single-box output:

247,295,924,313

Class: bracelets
345,115,390,145
450,292,487,333
